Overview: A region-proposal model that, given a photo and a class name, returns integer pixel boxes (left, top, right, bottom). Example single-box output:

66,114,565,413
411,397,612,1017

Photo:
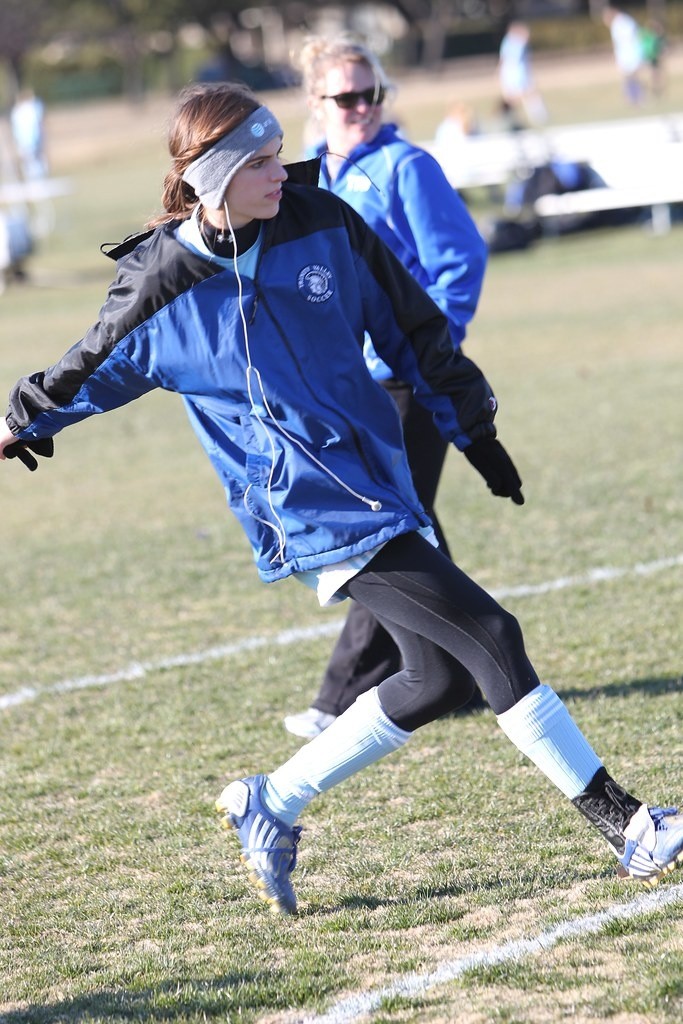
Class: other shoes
284,707,337,738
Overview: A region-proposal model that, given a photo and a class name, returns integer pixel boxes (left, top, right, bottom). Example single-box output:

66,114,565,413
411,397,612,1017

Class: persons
0,80,683,914
645,18,667,75
497,22,545,129
283,31,489,740
602,7,641,102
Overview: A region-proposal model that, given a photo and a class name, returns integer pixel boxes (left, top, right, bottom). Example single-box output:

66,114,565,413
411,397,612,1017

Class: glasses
322,84,384,108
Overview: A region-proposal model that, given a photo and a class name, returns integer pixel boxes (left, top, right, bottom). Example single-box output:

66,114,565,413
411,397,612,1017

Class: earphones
362,498,382,512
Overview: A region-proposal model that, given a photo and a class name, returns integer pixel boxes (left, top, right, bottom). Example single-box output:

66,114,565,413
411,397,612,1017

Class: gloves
3,436,53,471
464,437,524,505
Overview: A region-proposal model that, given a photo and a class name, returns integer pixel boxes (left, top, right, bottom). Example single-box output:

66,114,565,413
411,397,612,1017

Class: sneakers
608,804,683,889
215,774,303,914
